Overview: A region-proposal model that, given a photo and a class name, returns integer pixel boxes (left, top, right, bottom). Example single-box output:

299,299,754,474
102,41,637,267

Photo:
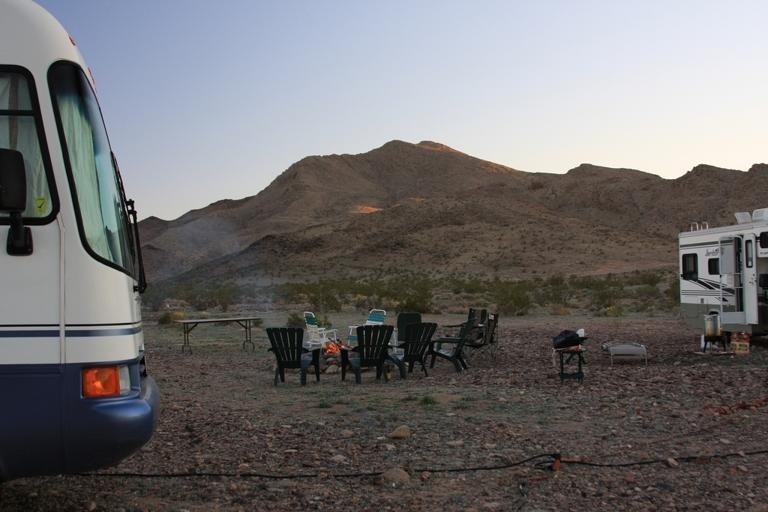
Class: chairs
265,308,498,386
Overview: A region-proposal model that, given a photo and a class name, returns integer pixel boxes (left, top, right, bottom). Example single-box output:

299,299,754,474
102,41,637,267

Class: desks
703,335,727,353
174,317,261,354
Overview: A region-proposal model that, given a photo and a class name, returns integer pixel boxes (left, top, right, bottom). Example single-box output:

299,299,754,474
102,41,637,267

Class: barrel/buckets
729,332,749,354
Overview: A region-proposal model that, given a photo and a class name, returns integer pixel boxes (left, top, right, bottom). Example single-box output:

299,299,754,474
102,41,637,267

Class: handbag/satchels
552,330,586,348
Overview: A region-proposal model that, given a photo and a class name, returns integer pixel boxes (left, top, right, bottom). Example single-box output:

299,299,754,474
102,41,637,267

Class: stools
556,347,588,385
610,342,648,366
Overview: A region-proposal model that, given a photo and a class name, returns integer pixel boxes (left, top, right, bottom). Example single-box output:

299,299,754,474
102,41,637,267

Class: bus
0,1,159,482
